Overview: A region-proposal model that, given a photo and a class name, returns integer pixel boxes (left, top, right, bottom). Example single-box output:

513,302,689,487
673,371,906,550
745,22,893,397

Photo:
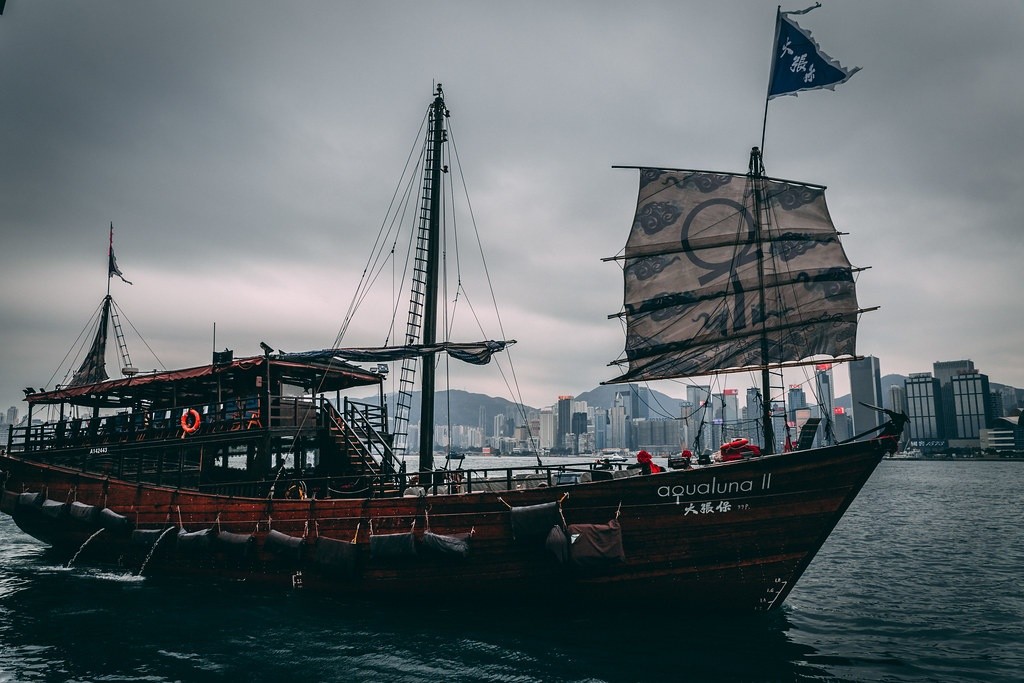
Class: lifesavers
181,409,200,432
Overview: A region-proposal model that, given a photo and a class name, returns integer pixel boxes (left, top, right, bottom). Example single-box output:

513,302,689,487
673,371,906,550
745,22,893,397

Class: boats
445,449,466,459
600,447,628,462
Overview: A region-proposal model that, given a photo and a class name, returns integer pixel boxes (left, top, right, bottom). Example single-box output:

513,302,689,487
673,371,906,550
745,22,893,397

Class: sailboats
1,1,909,654
